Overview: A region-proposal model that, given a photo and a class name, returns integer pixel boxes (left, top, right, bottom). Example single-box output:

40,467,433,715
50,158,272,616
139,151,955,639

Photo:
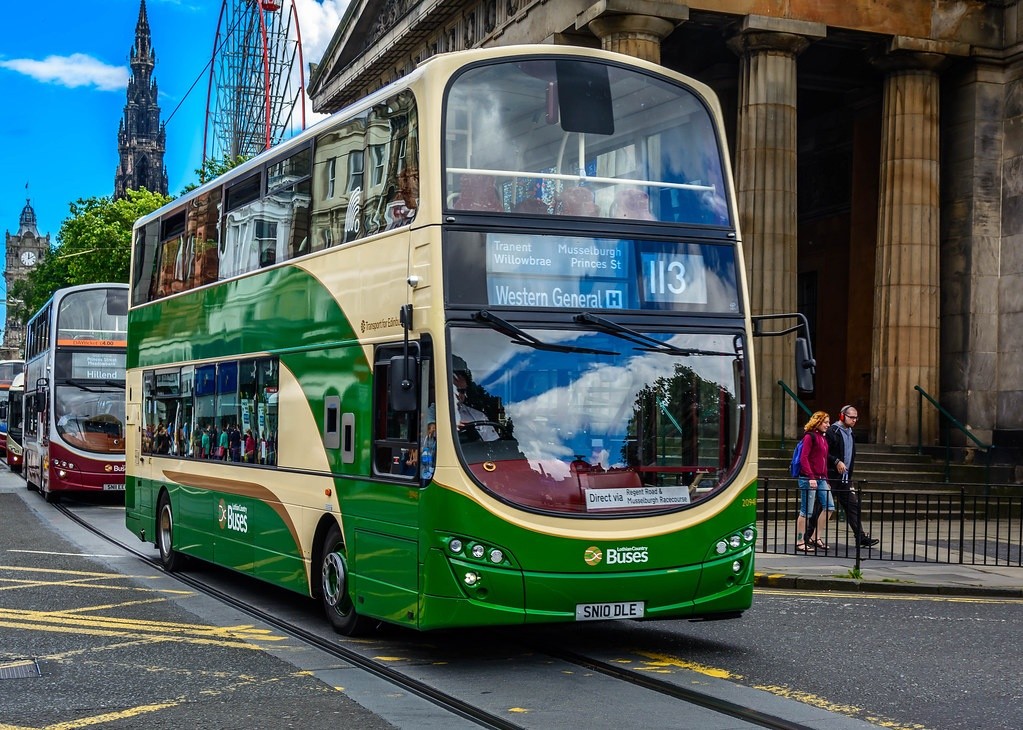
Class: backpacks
790,432,816,477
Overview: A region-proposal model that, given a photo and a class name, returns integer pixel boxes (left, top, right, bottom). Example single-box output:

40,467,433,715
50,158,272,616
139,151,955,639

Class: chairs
458,175,655,222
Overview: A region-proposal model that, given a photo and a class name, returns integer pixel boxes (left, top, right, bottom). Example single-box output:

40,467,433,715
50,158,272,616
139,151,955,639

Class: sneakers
856,536,879,548
804,530,814,544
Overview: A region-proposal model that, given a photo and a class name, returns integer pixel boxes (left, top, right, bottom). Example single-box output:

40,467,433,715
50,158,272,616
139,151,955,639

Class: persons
797,411,835,551
143,417,277,464
416,367,499,445
422,422,438,459
805,405,880,549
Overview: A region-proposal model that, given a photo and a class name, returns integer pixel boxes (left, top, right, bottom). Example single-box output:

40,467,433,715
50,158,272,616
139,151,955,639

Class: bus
0,372,24,473
22,281,131,503
0,360,25,457
123,44,817,642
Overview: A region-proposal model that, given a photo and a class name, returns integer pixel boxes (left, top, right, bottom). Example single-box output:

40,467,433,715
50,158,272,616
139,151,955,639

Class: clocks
20,251,37,266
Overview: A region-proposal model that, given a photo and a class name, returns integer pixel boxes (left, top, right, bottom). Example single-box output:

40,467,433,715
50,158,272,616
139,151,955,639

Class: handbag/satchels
173,444,177,452
201,448,205,457
189,446,194,455
218,446,224,456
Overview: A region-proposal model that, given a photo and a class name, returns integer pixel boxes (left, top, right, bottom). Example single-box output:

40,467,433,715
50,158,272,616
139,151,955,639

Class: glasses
457,389,468,395
844,413,858,421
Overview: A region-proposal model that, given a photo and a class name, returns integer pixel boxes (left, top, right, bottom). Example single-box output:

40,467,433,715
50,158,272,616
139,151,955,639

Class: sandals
808,536,830,550
796,543,815,552
